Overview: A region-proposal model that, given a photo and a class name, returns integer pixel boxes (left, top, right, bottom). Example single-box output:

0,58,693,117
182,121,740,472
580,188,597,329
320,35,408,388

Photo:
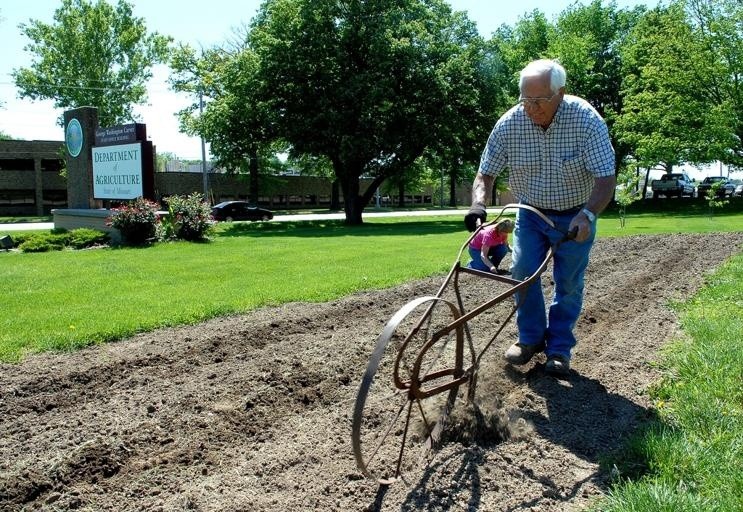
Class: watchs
582,208,595,222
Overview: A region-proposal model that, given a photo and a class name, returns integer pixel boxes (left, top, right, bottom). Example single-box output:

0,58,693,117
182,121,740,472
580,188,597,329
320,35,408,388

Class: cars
732,184,742,198
211,200,274,225
697,176,734,199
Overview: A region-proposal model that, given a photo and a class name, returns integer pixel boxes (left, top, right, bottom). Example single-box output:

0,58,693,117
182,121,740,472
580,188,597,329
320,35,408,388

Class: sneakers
545,354,571,376
503,339,547,366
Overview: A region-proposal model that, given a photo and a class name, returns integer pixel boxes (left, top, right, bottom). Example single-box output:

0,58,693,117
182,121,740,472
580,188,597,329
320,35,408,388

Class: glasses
518,93,557,106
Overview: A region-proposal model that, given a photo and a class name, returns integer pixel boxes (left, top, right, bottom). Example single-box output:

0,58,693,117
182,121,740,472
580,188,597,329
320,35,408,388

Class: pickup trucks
651,172,694,199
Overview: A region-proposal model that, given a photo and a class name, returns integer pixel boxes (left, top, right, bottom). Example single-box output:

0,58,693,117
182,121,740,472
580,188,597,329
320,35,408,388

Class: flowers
164,190,213,236
106,192,158,223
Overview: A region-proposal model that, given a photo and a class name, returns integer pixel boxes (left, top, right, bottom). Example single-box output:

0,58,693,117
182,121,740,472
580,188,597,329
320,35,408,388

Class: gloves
464,201,488,231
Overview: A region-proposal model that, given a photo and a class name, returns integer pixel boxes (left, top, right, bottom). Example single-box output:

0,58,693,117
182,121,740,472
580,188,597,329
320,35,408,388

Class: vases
175,225,197,240
124,222,154,246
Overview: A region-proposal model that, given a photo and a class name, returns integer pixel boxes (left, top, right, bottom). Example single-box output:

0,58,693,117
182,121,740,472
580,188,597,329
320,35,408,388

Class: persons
467,218,515,274
464,61,617,375
